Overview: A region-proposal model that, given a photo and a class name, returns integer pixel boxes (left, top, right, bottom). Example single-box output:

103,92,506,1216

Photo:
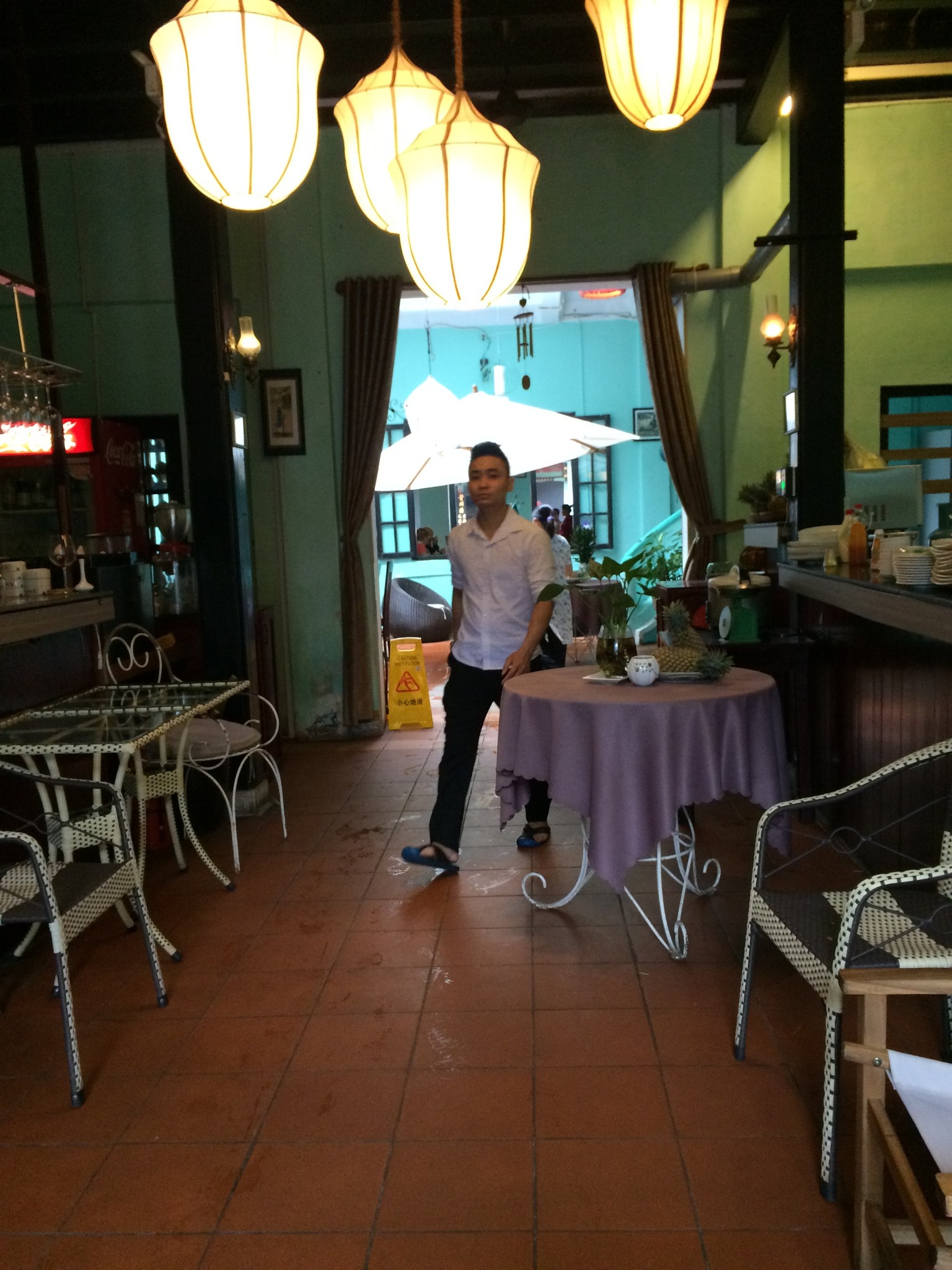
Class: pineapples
650,645,735,678
660,599,705,647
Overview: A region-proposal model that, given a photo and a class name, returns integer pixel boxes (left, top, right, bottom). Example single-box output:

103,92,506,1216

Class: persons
401,442,559,874
530,501,573,543
531,502,574,665
415,527,446,556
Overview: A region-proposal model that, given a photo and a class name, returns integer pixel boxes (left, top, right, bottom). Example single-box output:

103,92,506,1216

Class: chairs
733,740,952,1203
97,621,288,873
0,760,168,1108
834,968,952,1270
389,577,453,644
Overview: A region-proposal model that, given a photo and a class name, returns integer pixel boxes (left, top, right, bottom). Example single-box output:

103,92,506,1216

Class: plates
786,524,841,559
876,536,952,586
582,670,629,684
659,672,702,682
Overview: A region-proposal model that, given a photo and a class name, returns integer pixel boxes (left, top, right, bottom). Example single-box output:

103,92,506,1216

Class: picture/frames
258,368,307,456
633,407,662,441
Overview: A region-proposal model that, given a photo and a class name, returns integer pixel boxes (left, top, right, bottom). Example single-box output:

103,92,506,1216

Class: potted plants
569,525,599,577
535,550,647,678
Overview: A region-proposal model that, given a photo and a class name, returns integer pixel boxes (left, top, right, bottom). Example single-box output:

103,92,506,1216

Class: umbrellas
374,385,640,495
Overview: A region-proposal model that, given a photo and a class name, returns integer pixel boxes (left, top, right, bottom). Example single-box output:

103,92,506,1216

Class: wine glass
1,358,63,436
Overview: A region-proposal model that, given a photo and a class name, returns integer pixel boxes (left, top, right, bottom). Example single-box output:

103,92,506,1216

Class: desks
495,666,801,961
654,581,708,648
568,579,621,663
0,680,253,962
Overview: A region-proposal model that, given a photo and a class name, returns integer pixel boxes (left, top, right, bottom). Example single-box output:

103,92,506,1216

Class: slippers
516,823,551,846
401,843,461,872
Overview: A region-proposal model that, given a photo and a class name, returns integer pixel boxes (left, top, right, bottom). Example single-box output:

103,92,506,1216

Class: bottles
627,656,659,686
838,508,855,562
848,503,867,564
871,529,884,572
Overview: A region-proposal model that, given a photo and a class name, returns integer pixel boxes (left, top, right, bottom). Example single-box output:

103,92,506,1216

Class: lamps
149,0,730,311
760,295,796,369
223,315,262,388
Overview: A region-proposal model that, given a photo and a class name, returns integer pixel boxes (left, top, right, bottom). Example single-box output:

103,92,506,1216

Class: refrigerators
0,420,137,559
